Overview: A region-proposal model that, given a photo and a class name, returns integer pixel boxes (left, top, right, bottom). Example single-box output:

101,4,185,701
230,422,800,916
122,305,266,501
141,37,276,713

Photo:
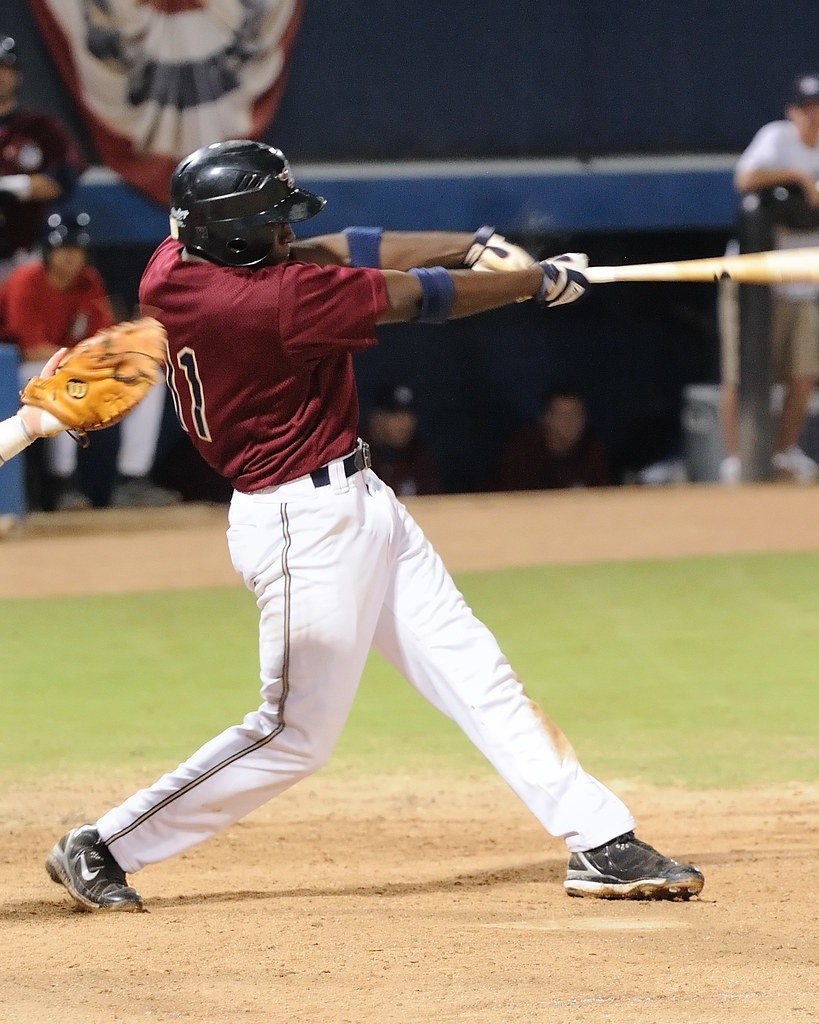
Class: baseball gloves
21,313,168,432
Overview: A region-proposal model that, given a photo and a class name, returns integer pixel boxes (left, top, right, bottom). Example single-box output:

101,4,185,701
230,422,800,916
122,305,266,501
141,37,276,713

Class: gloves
535,253,589,308
463,224,539,303
1,174,32,200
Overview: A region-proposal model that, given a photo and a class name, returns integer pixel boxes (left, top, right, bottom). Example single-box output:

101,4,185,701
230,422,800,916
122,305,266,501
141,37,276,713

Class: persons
0,33,87,265
716,74,819,485
499,394,614,489
0,318,167,473
0,217,183,510
362,377,445,495
42,141,705,915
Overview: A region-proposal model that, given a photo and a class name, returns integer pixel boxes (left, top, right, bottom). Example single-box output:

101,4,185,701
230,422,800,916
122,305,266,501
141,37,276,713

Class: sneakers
564,832,704,899
43,819,143,912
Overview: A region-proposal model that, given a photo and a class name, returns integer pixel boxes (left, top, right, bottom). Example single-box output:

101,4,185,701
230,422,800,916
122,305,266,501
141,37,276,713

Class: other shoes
55,487,94,511
109,477,180,507
772,445,818,477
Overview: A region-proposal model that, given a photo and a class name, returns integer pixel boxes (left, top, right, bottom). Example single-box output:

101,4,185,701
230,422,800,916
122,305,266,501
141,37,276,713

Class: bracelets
339,225,384,271
408,265,454,326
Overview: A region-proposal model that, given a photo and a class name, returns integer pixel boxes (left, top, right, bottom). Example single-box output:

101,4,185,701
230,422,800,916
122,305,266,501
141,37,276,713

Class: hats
375,383,418,410
787,70,819,103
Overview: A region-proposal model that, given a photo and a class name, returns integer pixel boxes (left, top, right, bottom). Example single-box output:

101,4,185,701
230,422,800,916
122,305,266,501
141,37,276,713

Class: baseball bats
589,246,819,292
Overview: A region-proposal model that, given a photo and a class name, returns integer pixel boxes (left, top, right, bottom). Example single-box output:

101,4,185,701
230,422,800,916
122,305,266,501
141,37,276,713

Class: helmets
169,140,328,267
39,214,91,268
0,34,18,69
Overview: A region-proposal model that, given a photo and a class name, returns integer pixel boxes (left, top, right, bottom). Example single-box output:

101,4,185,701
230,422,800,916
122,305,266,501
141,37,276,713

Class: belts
310,440,372,489
